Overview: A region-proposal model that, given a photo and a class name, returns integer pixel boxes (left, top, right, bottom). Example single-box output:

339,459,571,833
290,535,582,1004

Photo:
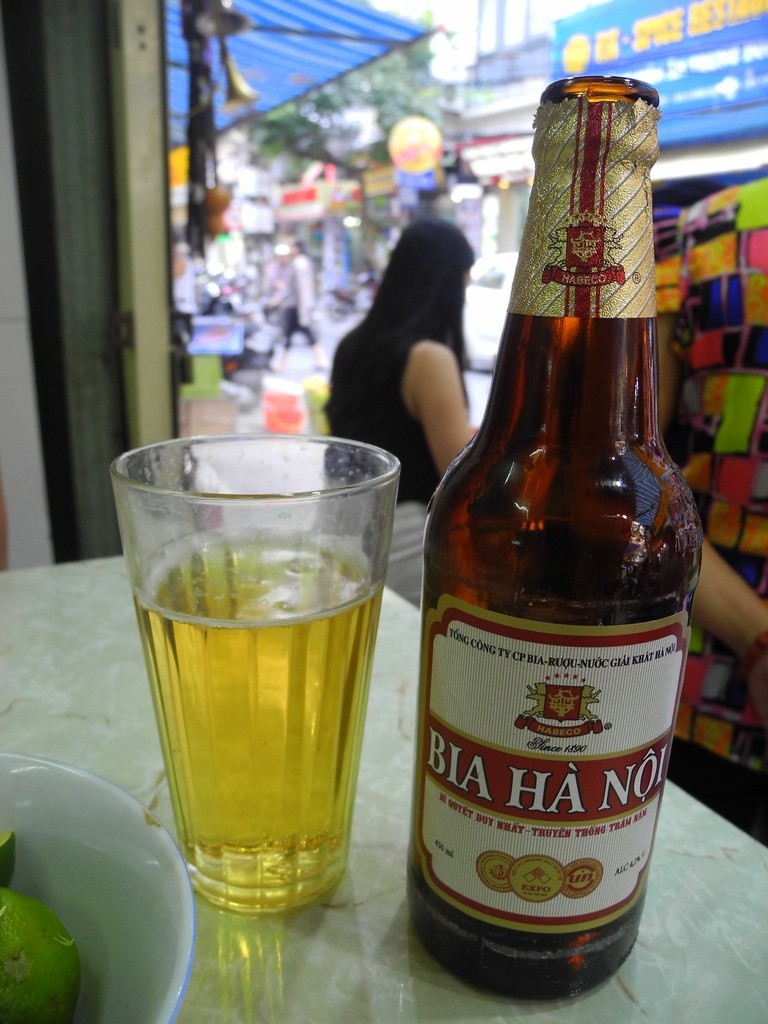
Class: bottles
410,74,708,1007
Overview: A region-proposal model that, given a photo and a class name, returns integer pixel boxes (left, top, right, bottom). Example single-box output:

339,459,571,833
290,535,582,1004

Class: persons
325,219,480,609
268,240,315,346
689,535,768,730
173,241,196,346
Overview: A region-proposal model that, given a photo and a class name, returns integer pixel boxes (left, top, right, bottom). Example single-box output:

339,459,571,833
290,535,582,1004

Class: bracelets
736,629,768,674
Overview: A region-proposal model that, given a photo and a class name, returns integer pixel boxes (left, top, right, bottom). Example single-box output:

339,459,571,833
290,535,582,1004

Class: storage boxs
170,254,246,437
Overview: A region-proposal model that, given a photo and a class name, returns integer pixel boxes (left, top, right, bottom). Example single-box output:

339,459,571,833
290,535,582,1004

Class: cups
110,431,404,917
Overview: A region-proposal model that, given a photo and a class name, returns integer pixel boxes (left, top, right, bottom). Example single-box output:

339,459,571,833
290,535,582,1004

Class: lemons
0,831,80,1024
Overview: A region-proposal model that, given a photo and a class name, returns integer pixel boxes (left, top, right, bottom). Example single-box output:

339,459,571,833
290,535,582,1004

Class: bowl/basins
0,751,197,1024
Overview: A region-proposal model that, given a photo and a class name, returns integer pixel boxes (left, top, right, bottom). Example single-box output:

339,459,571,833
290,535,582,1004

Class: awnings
165,0,436,150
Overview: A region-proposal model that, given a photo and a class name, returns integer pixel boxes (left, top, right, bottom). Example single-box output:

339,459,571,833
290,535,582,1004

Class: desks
0,554,768,1024
